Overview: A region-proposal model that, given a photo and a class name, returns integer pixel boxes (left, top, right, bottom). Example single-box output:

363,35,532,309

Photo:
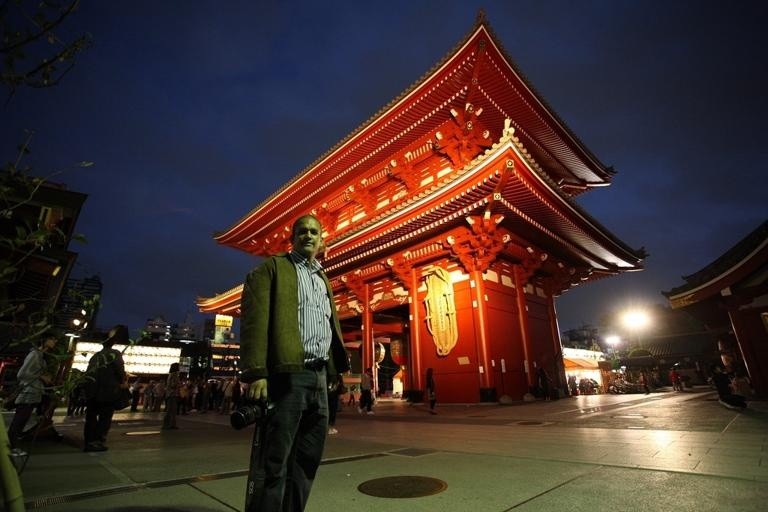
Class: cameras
229,395,279,430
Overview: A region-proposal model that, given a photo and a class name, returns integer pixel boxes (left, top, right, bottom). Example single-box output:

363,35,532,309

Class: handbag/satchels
114,388,132,410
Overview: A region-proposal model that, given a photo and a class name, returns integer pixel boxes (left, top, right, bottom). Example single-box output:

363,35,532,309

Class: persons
129,377,247,415
669,367,680,391
241,214,351,512
426,368,438,415
162,363,186,429
348,368,374,414
5,332,57,453
69,334,129,451
639,370,650,395
326,373,348,434
538,368,552,401
568,376,598,396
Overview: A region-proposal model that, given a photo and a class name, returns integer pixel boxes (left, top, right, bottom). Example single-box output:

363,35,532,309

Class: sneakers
87,441,109,451
10,448,28,456
367,411,375,415
328,428,338,434
357,406,362,414
